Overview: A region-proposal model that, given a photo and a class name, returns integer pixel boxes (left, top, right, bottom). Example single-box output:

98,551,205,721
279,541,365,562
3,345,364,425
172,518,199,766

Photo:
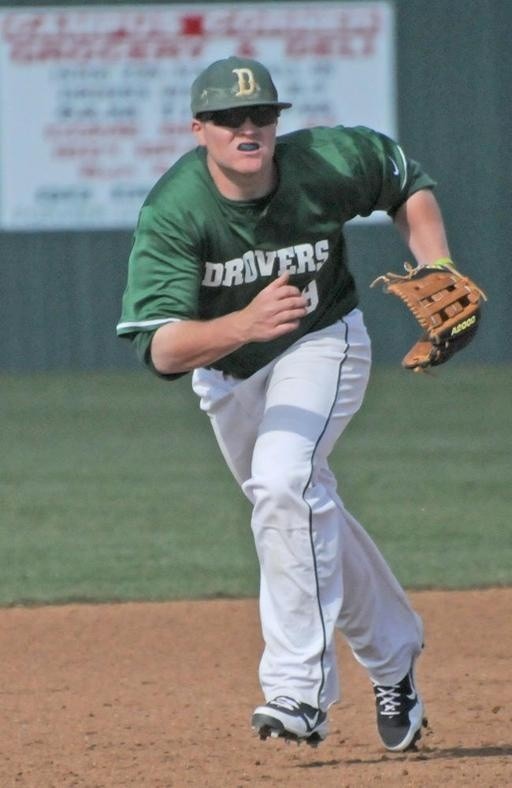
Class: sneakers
372,641,427,752
250,695,328,748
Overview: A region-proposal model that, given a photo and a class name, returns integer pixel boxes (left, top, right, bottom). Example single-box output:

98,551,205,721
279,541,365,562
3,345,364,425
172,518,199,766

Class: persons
116,55,488,753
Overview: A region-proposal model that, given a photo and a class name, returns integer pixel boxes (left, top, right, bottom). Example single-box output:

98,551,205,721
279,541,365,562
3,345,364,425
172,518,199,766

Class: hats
190,57,293,117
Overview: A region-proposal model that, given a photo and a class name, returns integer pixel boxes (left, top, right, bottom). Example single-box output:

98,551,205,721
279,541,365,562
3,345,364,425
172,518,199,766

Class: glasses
194,105,280,129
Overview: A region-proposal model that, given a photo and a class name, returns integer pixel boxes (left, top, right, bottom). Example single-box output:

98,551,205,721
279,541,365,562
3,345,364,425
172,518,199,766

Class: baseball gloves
370,257,486,373
